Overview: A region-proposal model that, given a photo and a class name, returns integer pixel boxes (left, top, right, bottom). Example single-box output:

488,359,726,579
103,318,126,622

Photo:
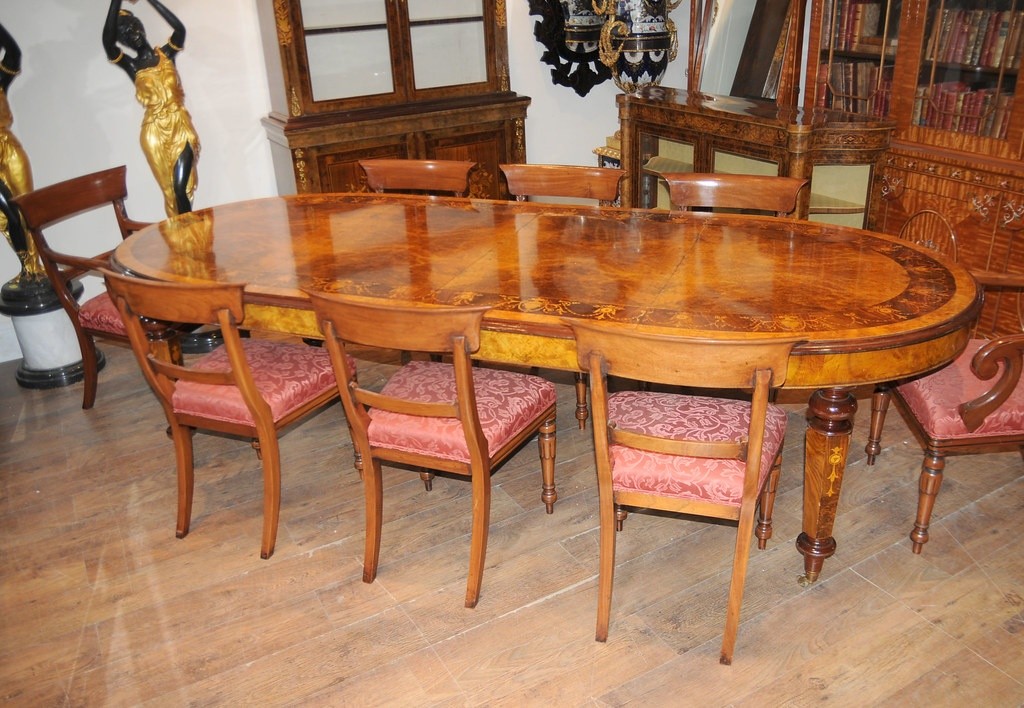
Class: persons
0,24,44,278
101,0,201,217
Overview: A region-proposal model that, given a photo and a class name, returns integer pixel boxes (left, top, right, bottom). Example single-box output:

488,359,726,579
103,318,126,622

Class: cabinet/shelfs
262,2,532,200
775,4,1024,340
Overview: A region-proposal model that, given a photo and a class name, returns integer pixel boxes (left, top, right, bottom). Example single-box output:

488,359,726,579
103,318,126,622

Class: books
816,0,903,121
912,5,1024,141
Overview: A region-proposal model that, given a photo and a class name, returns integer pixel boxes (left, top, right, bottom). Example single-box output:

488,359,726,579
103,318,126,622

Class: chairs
499,164,627,206
560,315,787,666
97,266,358,559
662,173,809,217
899,207,957,264
296,285,559,609
864,267,1024,555
355,158,477,198
12,165,251,440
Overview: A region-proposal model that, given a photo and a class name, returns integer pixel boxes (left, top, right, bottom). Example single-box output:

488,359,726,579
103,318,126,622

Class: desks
616,88,894,228
115,193,985,589
